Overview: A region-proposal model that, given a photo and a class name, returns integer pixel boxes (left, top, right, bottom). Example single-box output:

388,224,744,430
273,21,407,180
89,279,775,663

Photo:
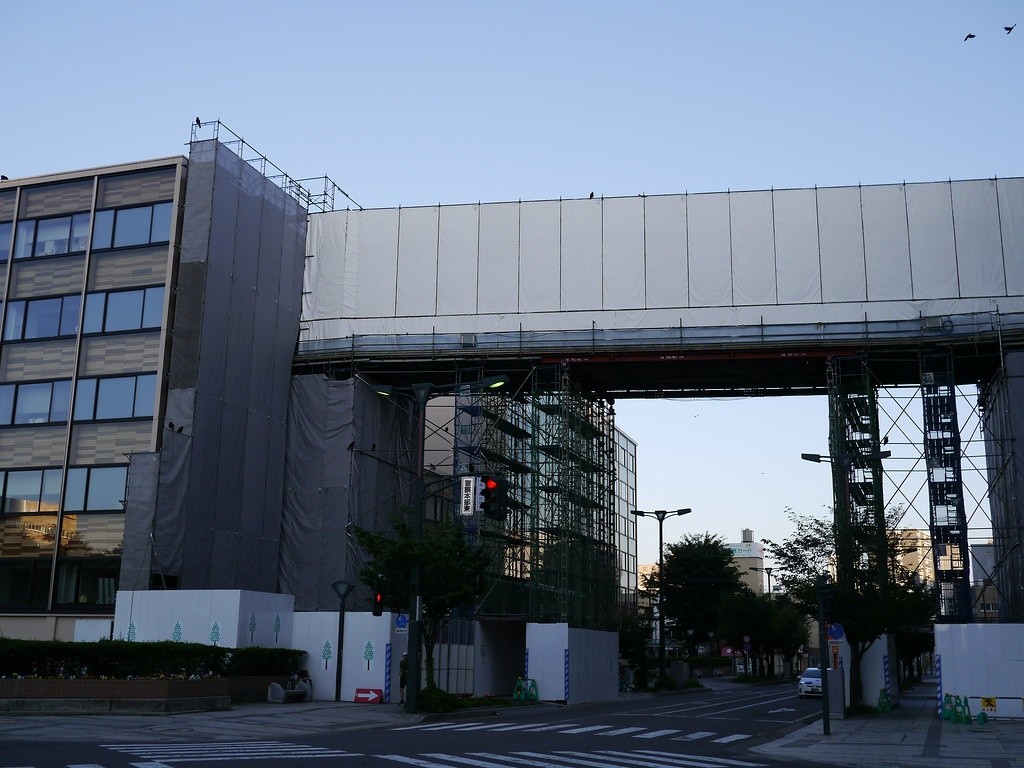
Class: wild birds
963,33,976,42
1002,24,1017,34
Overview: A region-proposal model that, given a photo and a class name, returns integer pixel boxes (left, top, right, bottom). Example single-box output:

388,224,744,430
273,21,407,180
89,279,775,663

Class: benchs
267,681,311,705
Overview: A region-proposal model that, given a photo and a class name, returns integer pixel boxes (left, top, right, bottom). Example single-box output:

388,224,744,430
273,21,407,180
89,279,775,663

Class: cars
796,667,834,698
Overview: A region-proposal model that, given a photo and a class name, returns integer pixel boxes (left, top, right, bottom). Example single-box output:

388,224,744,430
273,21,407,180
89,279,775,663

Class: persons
398,652,407,705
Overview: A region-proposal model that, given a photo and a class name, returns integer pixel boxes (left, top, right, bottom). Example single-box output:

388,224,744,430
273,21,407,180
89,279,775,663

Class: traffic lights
479,474,509,522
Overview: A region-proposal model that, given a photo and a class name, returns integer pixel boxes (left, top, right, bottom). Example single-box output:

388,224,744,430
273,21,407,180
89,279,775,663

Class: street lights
800,451,892,586
749,566,788,674
630,508,692,689
